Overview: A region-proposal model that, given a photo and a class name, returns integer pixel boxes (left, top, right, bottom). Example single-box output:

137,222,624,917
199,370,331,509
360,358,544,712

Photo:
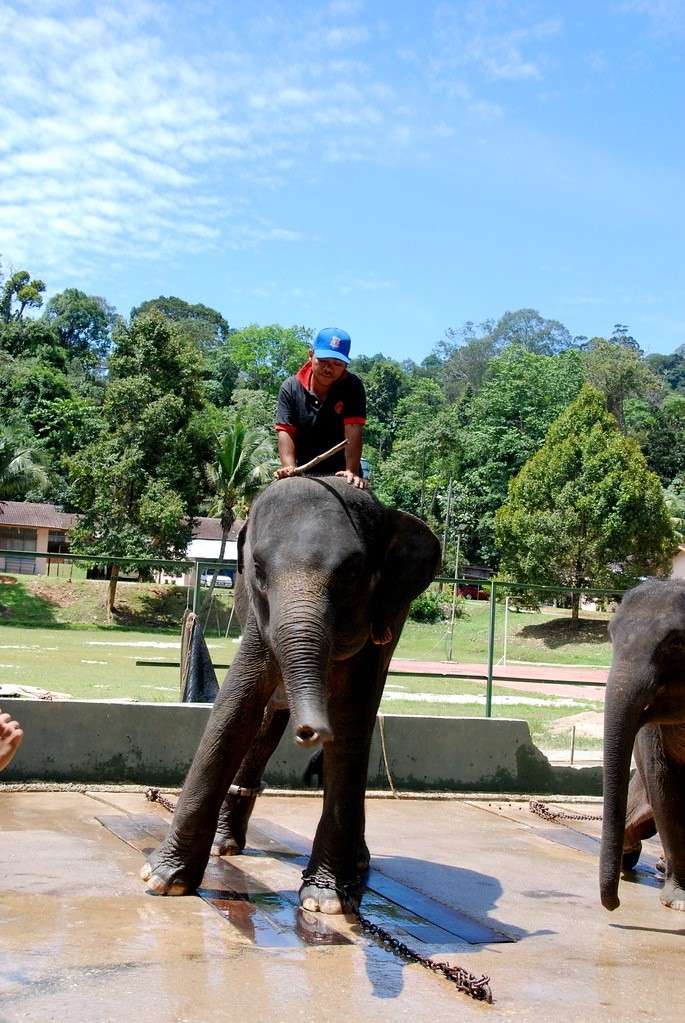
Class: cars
453,581,494,602
201,567,233,588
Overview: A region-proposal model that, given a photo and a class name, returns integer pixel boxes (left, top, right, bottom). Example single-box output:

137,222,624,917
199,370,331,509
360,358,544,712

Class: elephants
142,471,685,914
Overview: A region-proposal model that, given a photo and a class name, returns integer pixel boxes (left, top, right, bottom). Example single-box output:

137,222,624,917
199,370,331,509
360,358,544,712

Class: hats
313,328,351,364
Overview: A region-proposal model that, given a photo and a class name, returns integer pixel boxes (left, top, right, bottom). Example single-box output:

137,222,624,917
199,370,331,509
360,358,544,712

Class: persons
0,708,24,771
273,327,369,490
